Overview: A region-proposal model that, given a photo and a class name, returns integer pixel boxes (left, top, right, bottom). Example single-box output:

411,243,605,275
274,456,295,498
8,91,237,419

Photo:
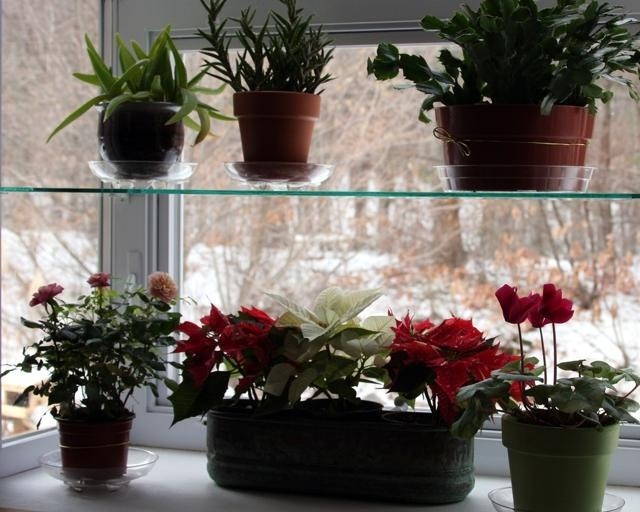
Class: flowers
0,270,199,428
167,289,530,417
449,284,640,427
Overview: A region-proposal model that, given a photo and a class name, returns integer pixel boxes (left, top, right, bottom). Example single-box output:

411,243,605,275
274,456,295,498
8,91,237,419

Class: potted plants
367,0,640,191
194,0,337,184
47,26,239,185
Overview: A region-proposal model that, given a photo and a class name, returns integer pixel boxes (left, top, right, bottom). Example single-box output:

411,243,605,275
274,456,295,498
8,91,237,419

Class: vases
272,397,382,500
502,412,620,512
53,414,136,478
204,396,276,494
380,408,477,505
